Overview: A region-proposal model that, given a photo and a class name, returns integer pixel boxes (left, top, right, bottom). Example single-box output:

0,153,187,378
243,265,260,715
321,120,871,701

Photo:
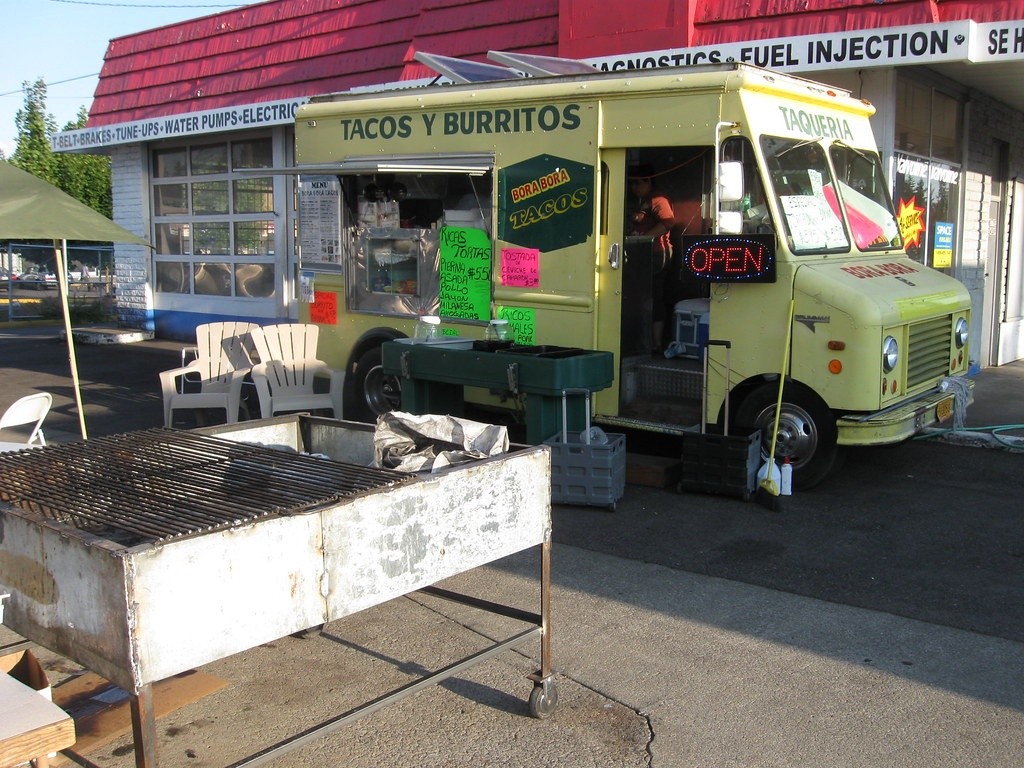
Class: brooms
753,300,794,514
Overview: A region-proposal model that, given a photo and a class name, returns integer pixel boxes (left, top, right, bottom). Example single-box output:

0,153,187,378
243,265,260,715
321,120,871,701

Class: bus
295,61,976,491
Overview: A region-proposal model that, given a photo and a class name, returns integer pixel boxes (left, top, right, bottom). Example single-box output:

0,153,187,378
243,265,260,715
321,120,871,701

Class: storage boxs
676,296,709,359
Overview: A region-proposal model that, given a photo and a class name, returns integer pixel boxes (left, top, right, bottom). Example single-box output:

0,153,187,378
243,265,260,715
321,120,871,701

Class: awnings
232,162,492,237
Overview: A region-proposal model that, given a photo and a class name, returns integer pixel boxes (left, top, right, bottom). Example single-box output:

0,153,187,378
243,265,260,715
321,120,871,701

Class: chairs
158,321,257,429
0,391,54,454
250,322,347,420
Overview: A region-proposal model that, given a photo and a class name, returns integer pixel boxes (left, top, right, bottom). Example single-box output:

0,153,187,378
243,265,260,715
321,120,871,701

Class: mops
858,376,969,432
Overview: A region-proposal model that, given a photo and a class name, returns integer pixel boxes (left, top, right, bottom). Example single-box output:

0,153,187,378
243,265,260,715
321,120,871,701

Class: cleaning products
756,455,792,496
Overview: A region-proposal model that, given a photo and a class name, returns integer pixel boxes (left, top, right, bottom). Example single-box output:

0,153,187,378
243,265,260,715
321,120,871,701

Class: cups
664,341,687,359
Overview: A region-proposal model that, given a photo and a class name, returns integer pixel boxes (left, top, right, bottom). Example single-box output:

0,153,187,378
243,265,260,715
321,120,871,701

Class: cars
0,266,74,290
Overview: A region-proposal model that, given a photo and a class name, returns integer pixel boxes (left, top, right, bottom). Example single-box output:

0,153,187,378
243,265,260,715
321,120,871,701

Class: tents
0,158,155,438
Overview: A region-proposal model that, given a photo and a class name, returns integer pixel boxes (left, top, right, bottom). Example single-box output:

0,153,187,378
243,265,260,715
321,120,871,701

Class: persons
81,263,89,280
627,164,675,350
68,272,73,282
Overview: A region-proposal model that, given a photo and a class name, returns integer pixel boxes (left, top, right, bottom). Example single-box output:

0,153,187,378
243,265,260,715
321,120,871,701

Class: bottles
485,319,514,342
414,316,443,343
579,426,608,453
781,456,792,496
756,458,781,495
